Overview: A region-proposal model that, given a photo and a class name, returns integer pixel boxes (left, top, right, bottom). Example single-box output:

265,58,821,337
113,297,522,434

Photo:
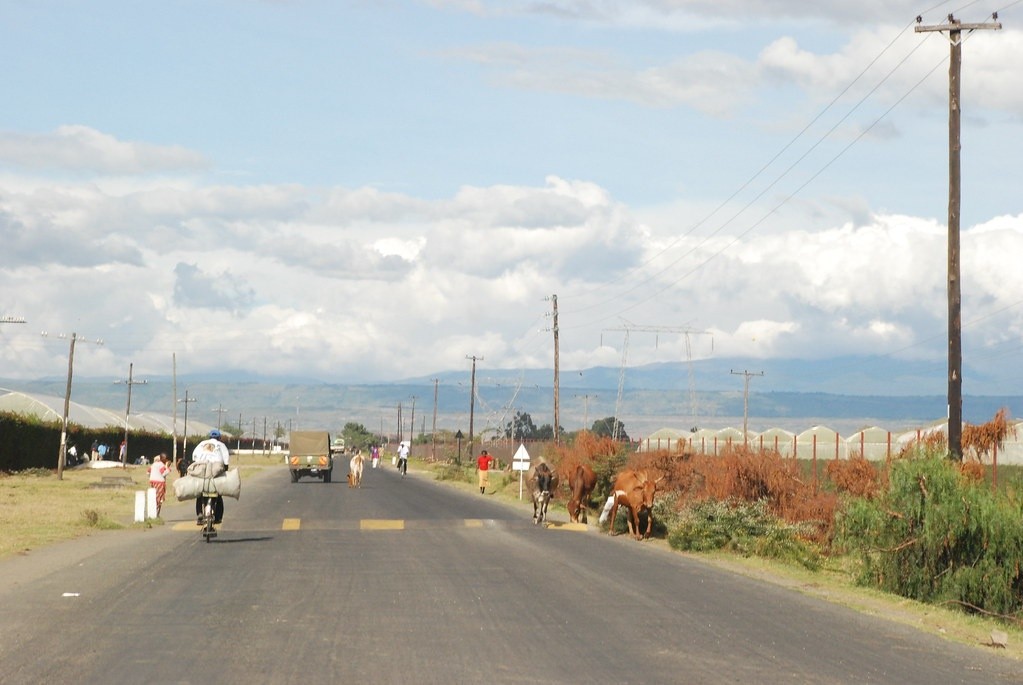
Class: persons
66,439,149,466
475,450,494,495
147,452,171,517
192,430,230,526
396,441,409,476
368,445,384,469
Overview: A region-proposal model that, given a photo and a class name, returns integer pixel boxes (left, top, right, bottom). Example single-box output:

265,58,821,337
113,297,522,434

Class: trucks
287,430,334,483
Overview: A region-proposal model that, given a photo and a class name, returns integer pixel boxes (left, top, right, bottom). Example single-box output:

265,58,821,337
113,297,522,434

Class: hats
209,429,221,439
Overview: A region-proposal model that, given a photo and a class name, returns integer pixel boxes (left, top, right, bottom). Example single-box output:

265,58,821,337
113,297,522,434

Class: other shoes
214,516,222,524
197,514,204,525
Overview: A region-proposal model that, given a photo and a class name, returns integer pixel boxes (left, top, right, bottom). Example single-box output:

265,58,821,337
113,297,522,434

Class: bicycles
397,457,406,478
197,493,221,542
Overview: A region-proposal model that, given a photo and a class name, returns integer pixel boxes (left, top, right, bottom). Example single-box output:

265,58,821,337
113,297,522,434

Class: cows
525,462,560,527
609,469,667,540
567,465,598,525
